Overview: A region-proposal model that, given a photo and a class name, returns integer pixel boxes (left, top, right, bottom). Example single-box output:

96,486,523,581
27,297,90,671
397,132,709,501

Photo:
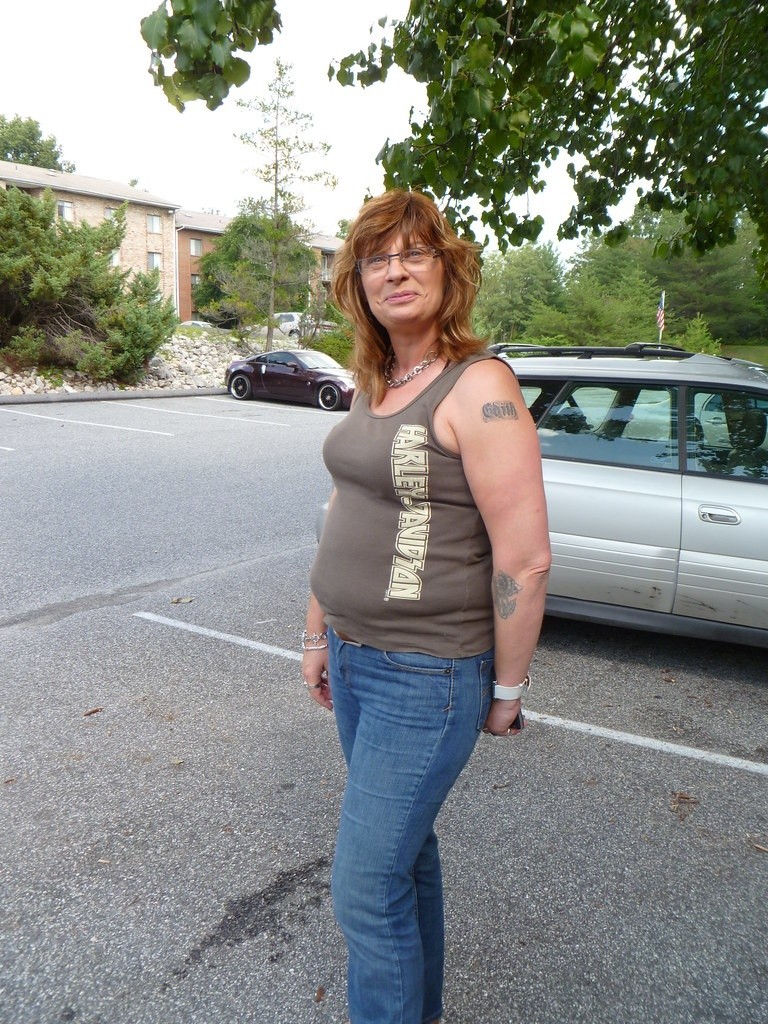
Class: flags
654,293,666,332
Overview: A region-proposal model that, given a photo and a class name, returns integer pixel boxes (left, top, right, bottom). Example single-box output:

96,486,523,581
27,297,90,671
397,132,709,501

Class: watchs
491,673,533,701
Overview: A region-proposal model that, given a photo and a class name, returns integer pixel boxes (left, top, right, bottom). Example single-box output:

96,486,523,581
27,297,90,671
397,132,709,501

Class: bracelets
298,627,329,651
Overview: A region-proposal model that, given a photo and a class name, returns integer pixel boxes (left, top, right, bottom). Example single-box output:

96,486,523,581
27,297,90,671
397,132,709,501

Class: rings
301,680,322,691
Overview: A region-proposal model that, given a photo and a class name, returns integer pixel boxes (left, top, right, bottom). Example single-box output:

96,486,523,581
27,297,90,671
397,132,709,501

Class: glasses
354,247,444,275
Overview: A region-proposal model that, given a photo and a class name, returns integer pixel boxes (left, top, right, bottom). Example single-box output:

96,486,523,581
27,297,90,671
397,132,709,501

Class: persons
293,187,558,1022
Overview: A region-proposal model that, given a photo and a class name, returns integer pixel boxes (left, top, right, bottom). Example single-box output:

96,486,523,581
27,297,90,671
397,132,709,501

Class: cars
240,311,336,341
513,382,768,452
224,349,361,412
482,338,768,657
180,320,215,329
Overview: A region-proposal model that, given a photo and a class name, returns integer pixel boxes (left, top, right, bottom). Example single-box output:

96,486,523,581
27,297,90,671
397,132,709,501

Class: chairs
673,415,724,474
725,409,767,479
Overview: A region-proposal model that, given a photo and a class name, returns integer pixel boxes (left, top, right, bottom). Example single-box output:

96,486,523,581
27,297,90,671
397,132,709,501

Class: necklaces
381,344,441,388
